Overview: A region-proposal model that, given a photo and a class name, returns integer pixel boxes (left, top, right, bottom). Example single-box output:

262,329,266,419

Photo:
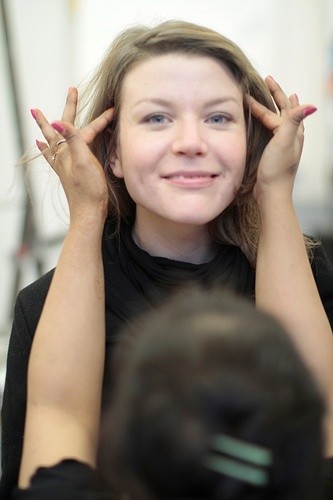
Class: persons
0,20,333,500
13,75,333,499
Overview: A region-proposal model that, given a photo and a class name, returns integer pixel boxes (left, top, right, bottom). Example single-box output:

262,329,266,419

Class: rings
51,152,57,161
57,140,66,146
